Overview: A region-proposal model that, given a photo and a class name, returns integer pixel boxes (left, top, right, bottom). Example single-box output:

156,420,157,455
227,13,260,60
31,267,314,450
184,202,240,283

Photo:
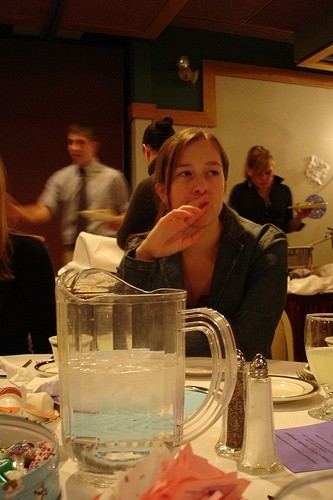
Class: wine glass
304,313,333,422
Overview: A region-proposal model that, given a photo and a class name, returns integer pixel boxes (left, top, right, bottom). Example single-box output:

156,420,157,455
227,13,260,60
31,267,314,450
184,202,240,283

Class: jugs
55,266,239,489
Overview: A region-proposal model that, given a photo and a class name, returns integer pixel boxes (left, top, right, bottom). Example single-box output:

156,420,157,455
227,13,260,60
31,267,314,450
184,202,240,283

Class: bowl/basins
0,414,60,500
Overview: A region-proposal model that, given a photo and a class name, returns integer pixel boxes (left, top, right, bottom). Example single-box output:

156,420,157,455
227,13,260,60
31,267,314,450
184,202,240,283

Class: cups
274,470,332,500
49,334,94,359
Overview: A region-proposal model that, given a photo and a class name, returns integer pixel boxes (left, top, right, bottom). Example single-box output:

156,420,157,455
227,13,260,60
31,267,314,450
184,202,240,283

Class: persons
0,158,60,357
0,118,313,272
113,126,289,362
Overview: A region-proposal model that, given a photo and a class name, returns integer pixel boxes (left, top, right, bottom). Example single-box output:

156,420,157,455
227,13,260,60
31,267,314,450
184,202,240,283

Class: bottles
238,354,283,476
215,348,247,461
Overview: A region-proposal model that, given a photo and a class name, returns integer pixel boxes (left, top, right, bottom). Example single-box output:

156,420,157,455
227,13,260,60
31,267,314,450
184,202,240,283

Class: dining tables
0,353,333,500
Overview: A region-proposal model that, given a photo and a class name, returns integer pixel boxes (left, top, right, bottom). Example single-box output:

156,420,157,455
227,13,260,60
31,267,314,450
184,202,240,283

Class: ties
77,166,89,241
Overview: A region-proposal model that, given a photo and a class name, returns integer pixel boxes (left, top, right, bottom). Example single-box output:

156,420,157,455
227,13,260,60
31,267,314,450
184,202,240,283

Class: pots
287,246,314,278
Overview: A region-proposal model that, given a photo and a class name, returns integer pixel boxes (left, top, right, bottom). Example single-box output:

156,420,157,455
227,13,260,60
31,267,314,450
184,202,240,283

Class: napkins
0,357,59,393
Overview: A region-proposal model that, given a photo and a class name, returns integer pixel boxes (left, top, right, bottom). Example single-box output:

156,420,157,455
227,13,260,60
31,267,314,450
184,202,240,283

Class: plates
34,360,55,377
270,374,320,401
78,209,123,223
288,202,329,209
40,414,60,429
184,356,213,376
